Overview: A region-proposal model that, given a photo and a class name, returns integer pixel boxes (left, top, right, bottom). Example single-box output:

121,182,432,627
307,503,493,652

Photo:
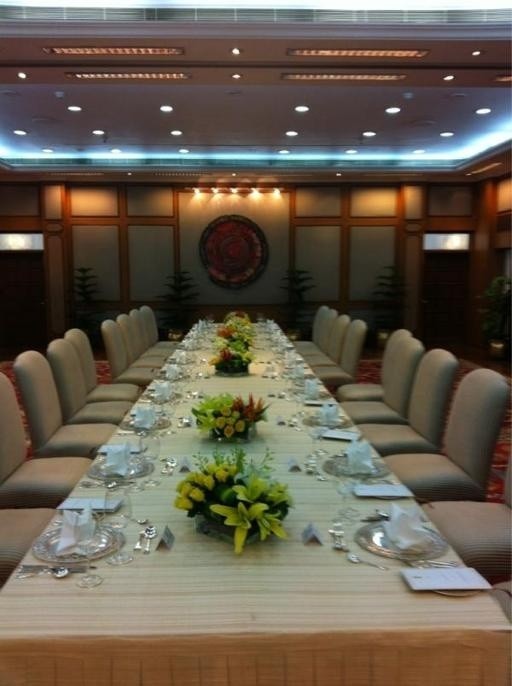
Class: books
300,397,337,407
127,407,169,418
56,495,126,517
352,479,416,504
95,441,149,456
321,425,365,444
396,566,496,594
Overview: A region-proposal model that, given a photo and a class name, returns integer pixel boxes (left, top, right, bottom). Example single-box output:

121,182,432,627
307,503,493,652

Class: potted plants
479,276,512,361
368,264,411,348
66,263,108,349
150,269,200,343
277,266,319,341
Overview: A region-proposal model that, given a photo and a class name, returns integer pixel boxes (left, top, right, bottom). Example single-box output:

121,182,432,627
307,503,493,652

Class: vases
215,363,249,373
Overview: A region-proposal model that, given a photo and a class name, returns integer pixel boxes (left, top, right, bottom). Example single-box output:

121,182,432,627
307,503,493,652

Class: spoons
80,480,120,490
135,530,146,550
15,568,70,581
346,552,389,573
168,455,176,477
144,525,157,556
328,517,348,551
121,510,149,526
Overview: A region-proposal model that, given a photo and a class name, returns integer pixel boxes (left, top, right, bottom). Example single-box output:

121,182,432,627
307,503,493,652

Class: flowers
208,311,257,365
190,392,272,438
175,446,296,555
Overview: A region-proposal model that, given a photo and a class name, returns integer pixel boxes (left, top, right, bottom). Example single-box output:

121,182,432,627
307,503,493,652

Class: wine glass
139,429,161,488
334,479,361,520
102,492,133,568
74,511,104,590
126,442,147,493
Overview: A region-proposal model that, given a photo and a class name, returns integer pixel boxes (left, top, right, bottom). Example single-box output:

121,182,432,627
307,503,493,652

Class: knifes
153,458,170,488
20,564,99,576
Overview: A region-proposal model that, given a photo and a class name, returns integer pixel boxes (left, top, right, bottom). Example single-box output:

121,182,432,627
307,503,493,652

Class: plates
117,317,218,439
253,317,391,482
354,480,404,500
88,455,156,481
418,562,482,598
32,523,126,564
352,520,449,561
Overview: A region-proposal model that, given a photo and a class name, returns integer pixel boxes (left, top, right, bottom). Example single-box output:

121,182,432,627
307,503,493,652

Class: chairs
376,365,511,503
47,336,133,435
351,347,460,455
11,350,120,462
291,306,367,388
102,306,183,387
64,326,140,404
490,581,511,622
416,466,512,582
335,329,424,423
0,371,93,579
